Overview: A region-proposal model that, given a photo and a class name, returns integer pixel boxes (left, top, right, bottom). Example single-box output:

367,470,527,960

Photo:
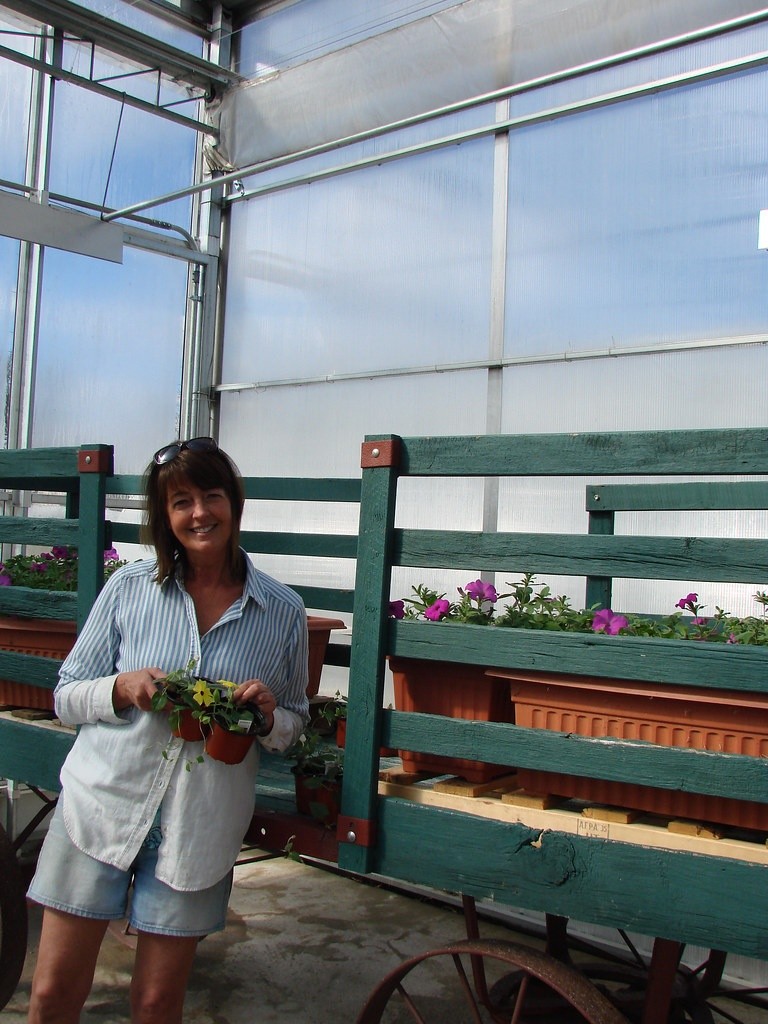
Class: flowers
150,659,253,773
388,572,768,646
0,546,143,591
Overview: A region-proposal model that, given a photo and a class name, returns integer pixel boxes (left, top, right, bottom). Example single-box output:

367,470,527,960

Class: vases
206,696,266,765
0,617,76,711
163,676,222,742
306,616,347,698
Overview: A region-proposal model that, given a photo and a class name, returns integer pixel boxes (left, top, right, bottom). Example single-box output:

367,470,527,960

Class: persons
27,437,312,1024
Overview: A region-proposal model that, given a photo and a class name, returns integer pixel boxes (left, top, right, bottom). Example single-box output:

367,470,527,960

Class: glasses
153,437,226,472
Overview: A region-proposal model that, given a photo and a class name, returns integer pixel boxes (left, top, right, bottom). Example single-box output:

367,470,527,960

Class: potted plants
312,689,348,748
386,656,516,783
291,729,344,829
485,668,768,831
